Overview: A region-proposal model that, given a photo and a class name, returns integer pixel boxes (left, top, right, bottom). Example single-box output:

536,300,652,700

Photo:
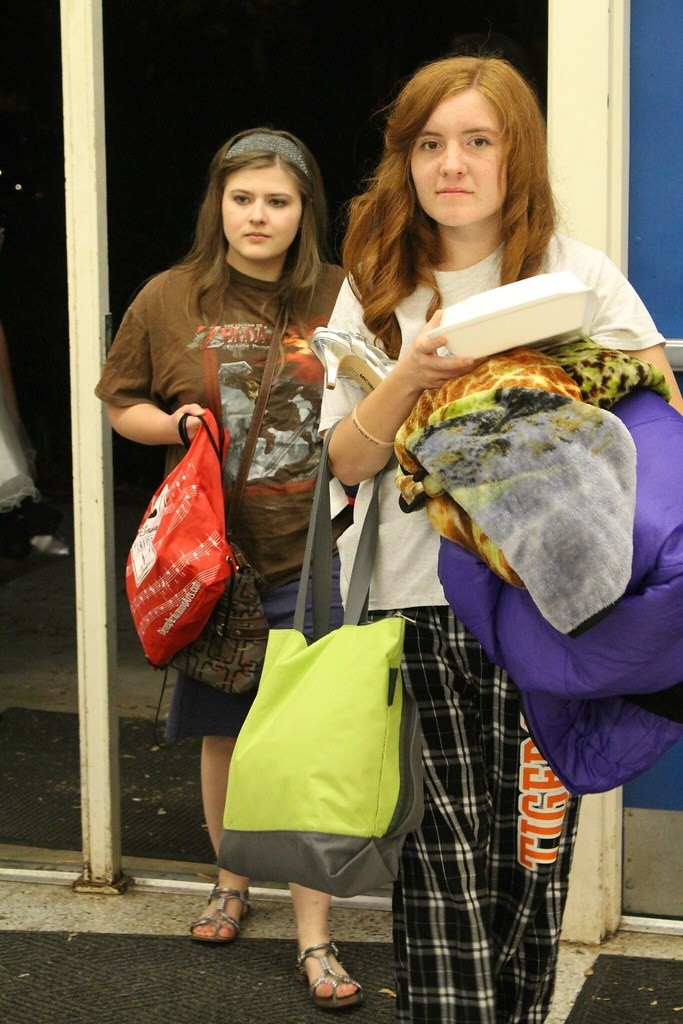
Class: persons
315,56,682,1024
96,129,365,1007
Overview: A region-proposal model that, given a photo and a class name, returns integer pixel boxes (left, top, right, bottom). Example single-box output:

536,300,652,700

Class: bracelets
351,401,395,449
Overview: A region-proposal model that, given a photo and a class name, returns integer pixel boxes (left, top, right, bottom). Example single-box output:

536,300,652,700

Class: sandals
191,882,250,941
296,940,364,1007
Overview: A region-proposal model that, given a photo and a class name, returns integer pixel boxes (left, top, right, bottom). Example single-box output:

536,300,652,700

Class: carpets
0,707,218,863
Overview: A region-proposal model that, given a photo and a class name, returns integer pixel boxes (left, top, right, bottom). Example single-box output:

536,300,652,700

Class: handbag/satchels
125,408,237,667
217,617,424,898
173,542,268,694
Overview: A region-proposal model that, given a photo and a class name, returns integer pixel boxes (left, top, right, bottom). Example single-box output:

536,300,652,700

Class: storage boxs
427,270,598,357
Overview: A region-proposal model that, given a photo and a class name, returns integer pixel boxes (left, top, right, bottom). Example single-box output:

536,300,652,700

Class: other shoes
31,535,69,555
